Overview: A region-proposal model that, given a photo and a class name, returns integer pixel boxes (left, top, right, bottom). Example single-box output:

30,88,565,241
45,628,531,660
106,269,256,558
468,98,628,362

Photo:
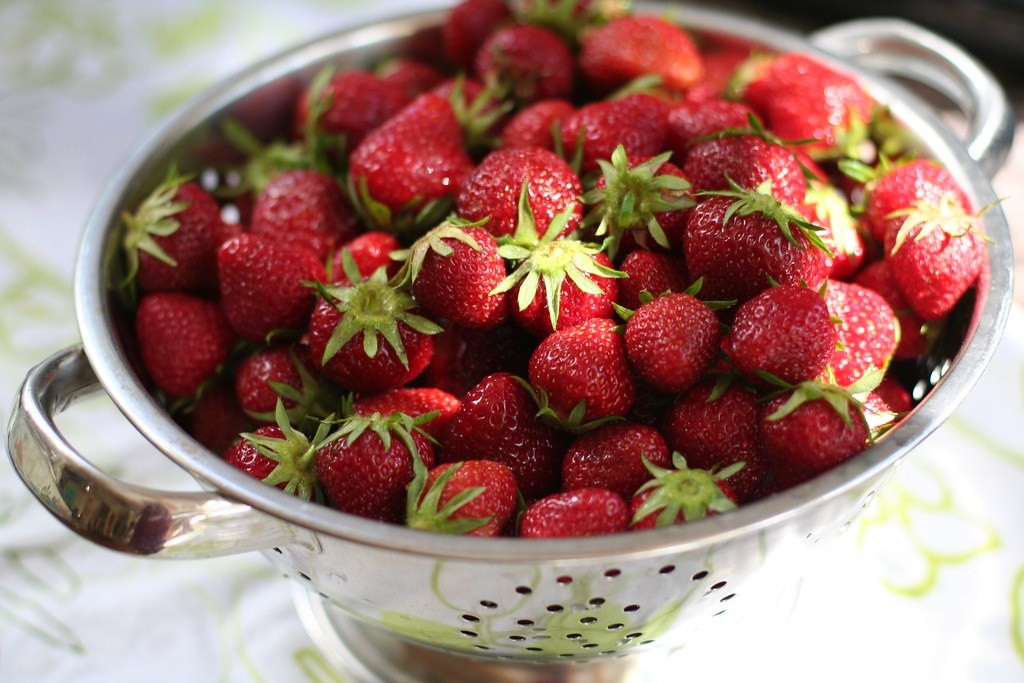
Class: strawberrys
121,0,993,538
